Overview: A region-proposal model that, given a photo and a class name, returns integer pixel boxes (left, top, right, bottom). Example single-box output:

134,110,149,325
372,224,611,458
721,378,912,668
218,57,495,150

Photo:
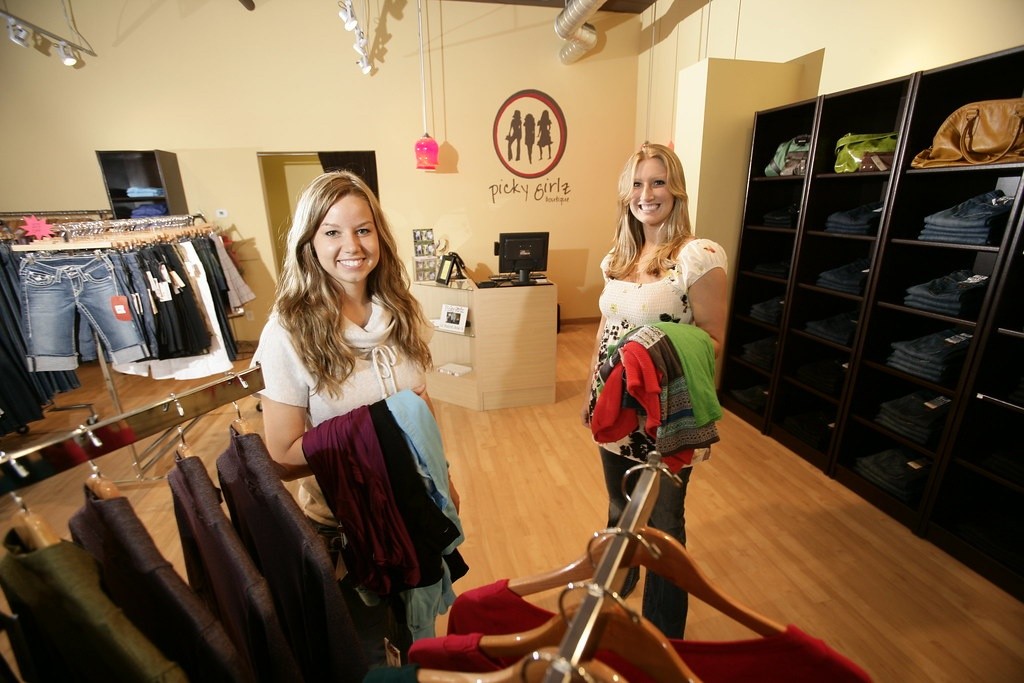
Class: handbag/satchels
764,135,812,176
834,131,899,174
910,98,1024,167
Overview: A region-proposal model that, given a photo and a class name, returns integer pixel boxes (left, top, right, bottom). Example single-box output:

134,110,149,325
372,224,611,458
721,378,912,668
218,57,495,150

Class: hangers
0,205,873,683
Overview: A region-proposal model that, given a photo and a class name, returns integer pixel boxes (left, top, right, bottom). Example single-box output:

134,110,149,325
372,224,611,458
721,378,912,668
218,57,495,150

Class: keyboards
489,273,548,281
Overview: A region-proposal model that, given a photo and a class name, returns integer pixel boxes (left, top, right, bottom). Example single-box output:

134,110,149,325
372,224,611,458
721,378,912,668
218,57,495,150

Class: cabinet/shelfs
674,43,1024,608
94,147,189,219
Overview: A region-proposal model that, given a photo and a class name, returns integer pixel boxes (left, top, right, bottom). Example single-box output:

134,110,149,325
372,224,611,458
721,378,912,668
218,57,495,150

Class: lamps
338,0,374,75
414,0,439,171
0,0,84,67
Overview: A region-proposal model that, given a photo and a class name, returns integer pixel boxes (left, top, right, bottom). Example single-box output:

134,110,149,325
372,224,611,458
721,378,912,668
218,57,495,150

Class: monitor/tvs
498,232,549,285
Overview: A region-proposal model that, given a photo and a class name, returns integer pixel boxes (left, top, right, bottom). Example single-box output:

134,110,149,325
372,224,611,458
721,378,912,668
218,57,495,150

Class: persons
250,171,460,663
580,143,728,641
